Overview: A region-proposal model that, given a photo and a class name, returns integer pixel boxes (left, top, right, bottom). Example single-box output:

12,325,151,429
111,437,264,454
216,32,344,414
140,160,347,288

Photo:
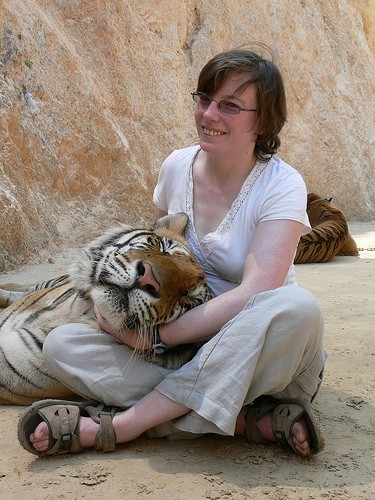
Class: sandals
17,400,124,458
244,393,325,458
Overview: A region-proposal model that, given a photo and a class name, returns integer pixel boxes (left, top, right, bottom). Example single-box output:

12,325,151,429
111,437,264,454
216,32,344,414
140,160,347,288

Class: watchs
148,325,167,355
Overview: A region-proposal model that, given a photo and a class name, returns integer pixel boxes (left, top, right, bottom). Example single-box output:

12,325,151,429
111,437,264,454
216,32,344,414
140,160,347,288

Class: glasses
191,91,257,115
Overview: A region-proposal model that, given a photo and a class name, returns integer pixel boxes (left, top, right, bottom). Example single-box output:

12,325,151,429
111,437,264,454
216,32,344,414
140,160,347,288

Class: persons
16,41,328,459
